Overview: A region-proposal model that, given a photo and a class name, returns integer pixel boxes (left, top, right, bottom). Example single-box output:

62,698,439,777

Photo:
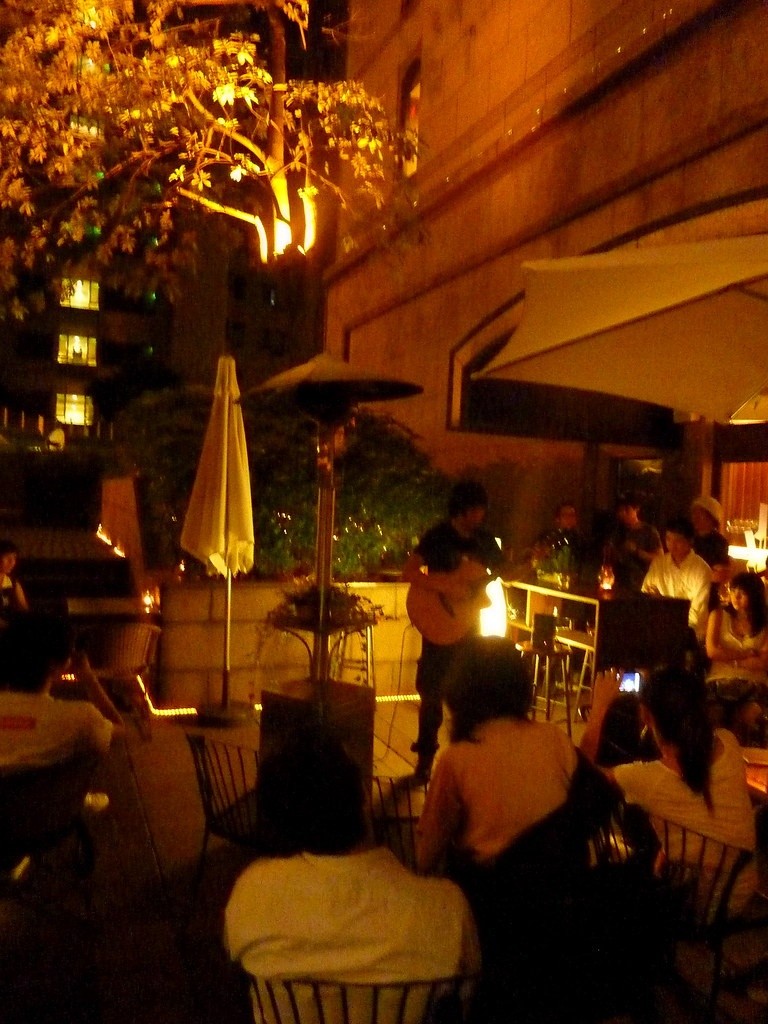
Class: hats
689,496,727,535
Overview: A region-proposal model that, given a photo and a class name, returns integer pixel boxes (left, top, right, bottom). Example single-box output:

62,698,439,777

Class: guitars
405,527,582,647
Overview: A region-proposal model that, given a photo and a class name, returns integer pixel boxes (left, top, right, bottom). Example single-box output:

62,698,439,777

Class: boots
412,748,436,785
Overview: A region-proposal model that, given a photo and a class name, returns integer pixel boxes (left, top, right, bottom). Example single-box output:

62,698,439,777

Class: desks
508,578,692,710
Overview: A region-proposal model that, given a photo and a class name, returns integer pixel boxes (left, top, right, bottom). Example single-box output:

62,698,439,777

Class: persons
577,666,759,931
219,738,482,1024
0,539,30,617
400,481,505,790
531,492,768,746
412,635,618,979
0,608,127,889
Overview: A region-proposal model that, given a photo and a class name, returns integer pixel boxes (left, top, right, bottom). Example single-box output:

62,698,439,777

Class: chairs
0,734,753,1024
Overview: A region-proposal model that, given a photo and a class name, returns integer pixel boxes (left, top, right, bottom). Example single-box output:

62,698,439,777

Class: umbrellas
180,346,256,709
467,232,768,423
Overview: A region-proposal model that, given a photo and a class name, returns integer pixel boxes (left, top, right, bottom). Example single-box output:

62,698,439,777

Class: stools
516,641,574,742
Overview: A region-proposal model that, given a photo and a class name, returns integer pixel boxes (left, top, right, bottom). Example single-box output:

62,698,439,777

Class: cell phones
616,672,642,692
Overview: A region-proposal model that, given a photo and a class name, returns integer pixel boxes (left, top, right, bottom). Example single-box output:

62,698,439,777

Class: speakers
531,614,557,649
